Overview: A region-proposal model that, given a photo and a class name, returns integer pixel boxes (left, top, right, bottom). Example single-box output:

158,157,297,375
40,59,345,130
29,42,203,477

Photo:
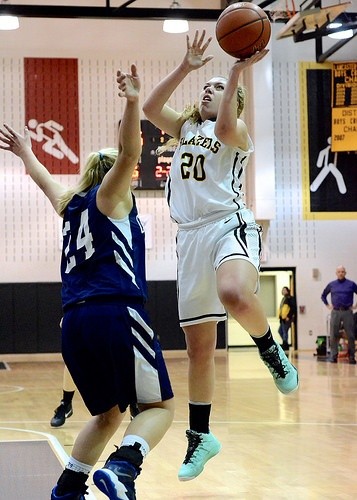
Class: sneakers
177,429,223,480
50,485,86,500
93,458,137,500
257,339,300,394
50,399,73,427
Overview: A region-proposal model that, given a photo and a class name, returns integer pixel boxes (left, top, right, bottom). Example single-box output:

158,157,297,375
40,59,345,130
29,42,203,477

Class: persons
50,317,140,427
321,265,357,364
277,287,296,351
141,29,299,482
0,63,175,500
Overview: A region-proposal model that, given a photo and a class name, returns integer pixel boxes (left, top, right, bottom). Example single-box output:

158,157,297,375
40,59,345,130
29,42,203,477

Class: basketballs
215,1,272,59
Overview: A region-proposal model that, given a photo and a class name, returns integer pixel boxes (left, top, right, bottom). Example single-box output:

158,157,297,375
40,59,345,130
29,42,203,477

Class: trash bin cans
316,336,327,355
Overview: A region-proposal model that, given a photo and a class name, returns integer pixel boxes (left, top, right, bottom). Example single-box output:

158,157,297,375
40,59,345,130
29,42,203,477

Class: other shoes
348,358,357,364
329,357,337,363
